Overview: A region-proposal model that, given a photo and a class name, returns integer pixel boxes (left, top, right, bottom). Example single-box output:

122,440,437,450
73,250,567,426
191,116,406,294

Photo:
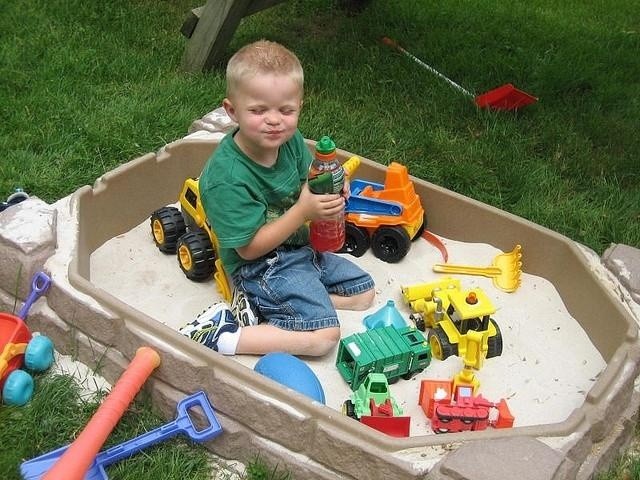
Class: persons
175,37,378,358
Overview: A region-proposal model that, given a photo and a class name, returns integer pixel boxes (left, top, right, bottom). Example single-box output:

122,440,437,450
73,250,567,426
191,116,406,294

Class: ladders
180,0,375,75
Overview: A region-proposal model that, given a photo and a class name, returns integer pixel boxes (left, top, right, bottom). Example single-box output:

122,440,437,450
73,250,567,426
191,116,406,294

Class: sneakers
229,285,263,329
178,300,239,353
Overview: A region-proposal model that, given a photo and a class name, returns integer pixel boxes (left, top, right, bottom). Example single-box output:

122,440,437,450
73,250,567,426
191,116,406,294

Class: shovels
19,391,223,480
382,37,539,112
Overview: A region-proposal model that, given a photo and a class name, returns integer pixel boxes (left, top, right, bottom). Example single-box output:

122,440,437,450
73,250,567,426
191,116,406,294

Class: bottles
307,136,346,252
